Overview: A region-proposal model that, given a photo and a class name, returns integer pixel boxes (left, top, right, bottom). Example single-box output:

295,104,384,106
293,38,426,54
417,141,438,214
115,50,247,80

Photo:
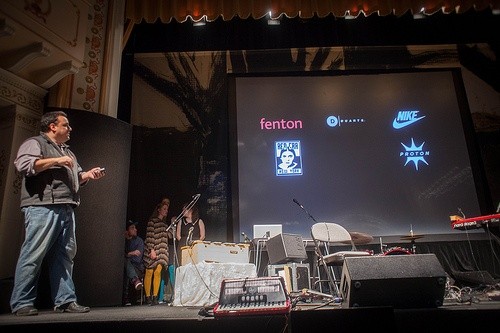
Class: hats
126,219,138,228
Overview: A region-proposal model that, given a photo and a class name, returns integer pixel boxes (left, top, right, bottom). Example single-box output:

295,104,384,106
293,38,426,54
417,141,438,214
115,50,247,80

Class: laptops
253,224,282,239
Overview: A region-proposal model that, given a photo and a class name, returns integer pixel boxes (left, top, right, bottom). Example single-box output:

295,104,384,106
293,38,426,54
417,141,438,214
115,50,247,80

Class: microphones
192,194,200,198
293,198,304,209
241,232,251,242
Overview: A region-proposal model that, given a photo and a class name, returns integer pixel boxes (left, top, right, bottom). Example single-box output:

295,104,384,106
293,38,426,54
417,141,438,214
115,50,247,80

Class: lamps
193,18,206,26
267,11,281,25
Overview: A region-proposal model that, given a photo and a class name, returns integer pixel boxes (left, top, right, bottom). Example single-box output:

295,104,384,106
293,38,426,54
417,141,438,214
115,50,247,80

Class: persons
9,111,106,317
123,220,144,306
156,198,172,224
167,204,206,307
143,203,177,307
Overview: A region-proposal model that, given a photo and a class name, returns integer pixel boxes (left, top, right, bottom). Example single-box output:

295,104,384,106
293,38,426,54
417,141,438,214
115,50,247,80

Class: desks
175,263,256,308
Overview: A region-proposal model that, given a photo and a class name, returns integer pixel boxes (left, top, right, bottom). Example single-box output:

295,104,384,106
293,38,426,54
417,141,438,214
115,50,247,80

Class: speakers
340,253,447,308
266,233,308,264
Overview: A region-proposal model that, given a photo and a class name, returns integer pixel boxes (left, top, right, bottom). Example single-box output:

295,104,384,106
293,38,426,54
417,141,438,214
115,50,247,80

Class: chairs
311,222,369,296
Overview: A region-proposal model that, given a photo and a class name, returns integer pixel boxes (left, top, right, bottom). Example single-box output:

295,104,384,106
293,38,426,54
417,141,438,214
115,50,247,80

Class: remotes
99,168,105,173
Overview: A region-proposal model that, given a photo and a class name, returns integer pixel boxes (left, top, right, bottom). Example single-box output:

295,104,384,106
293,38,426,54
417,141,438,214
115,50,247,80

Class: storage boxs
267,262,311,292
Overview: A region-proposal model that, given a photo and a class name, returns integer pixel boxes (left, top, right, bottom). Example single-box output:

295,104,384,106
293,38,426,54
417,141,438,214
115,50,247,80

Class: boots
130,278,143,290
153,296,158,305
122,294,131,305
146,296,154,306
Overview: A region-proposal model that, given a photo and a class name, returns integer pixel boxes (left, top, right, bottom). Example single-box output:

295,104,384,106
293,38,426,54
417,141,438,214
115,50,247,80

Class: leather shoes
54,301,90,313
16,306,39,316
